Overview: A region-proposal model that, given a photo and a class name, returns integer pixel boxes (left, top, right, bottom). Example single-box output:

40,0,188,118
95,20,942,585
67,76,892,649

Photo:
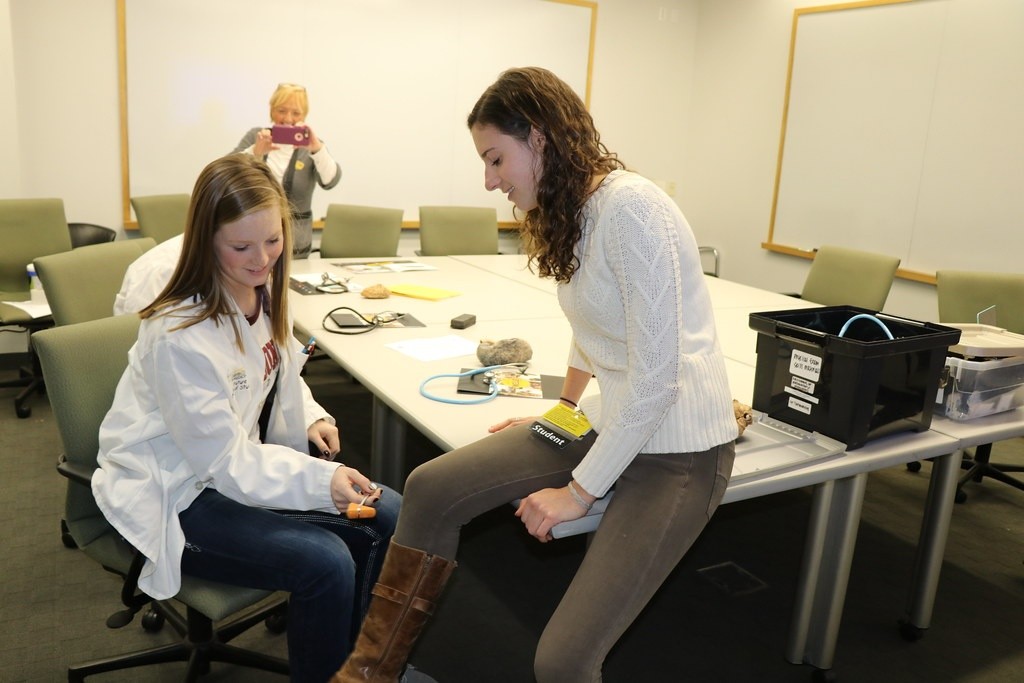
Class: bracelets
567,481,591,510
560,397,577,407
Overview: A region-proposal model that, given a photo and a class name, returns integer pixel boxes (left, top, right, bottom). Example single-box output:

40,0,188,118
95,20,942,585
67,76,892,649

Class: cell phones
272,126,309,145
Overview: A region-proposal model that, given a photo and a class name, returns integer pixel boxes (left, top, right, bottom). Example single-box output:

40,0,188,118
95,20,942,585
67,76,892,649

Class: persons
333,67,741,683
223,82,342,377
112,230,204,317
90,155,404,683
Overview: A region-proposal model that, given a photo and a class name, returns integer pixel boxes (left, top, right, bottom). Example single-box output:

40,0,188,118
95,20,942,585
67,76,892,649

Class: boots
326,532,457,683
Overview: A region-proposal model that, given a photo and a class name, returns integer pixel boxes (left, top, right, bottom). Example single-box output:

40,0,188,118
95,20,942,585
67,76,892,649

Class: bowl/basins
351,270,399,299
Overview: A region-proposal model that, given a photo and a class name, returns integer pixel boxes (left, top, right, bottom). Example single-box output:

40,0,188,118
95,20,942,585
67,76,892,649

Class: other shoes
400,663,438,683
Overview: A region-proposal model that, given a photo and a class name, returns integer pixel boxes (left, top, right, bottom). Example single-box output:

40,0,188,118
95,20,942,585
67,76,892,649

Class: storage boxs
931,323,1024,422
748,304,962,452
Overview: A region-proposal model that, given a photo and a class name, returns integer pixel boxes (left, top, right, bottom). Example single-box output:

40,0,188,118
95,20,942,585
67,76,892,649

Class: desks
454,256,1024,644
285,256,959,683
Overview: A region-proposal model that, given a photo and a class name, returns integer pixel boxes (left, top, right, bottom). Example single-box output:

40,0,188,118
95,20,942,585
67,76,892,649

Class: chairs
299,205,404,384
905,268,1024,503
32,237,158,548
418,205,500,256
779,242,901,311
0,196,74,419
30,312,292,683
130,193,191,244
